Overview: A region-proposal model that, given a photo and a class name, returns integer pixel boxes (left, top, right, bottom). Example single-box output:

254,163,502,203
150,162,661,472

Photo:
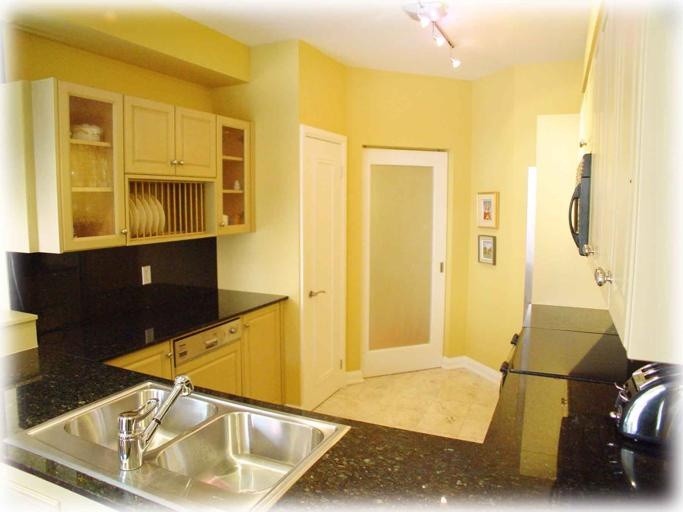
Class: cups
224,214,228,226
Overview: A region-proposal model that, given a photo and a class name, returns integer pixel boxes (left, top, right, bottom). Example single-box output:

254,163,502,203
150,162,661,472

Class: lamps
406,1,462,71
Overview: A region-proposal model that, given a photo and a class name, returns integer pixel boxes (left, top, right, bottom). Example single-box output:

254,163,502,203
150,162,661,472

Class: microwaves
568,154,591,256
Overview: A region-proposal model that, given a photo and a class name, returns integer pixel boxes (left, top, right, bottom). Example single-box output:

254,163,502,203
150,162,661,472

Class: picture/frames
478,235,496,266
477,191,500,229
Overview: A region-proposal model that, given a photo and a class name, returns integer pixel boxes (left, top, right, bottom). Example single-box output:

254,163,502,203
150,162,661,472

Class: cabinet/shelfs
102,340,172,381
0,77,126,256
582,0,683,367
172,317,244,397
242,301,285,406
216,114,254,235
122,95,216,247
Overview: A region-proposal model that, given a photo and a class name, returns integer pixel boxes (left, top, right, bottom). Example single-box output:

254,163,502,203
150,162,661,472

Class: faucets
118,375,193,470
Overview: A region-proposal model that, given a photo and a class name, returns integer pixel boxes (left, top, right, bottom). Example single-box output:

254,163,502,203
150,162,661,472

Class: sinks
7,381,220,454
152,410,350,512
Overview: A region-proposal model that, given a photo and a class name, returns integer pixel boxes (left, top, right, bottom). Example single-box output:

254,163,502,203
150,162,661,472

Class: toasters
610,360,681,448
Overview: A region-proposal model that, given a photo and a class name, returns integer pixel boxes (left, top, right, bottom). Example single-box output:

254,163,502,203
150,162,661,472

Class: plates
128,192,165,234
